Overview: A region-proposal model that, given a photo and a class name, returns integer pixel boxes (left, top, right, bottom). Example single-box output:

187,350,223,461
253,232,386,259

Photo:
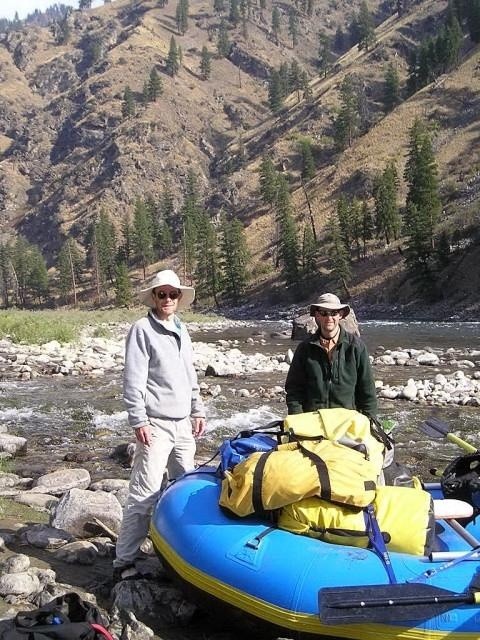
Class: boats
150,466,480,640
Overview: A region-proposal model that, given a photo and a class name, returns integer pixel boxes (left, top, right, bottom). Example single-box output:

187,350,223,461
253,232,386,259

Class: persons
284,293,378,427
112,269,207,581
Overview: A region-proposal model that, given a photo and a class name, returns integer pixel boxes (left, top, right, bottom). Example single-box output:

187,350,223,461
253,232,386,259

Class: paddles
416,416,477,453
317,581,480,625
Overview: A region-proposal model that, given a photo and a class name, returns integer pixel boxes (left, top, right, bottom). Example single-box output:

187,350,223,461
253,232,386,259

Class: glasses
156,291,181,299
316,309,339,316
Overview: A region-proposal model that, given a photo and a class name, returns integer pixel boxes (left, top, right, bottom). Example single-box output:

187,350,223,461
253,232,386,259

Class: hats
138,269,195,310
310,292,350,319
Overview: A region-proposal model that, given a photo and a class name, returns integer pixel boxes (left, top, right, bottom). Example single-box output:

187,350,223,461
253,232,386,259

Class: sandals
113,564,145,582
